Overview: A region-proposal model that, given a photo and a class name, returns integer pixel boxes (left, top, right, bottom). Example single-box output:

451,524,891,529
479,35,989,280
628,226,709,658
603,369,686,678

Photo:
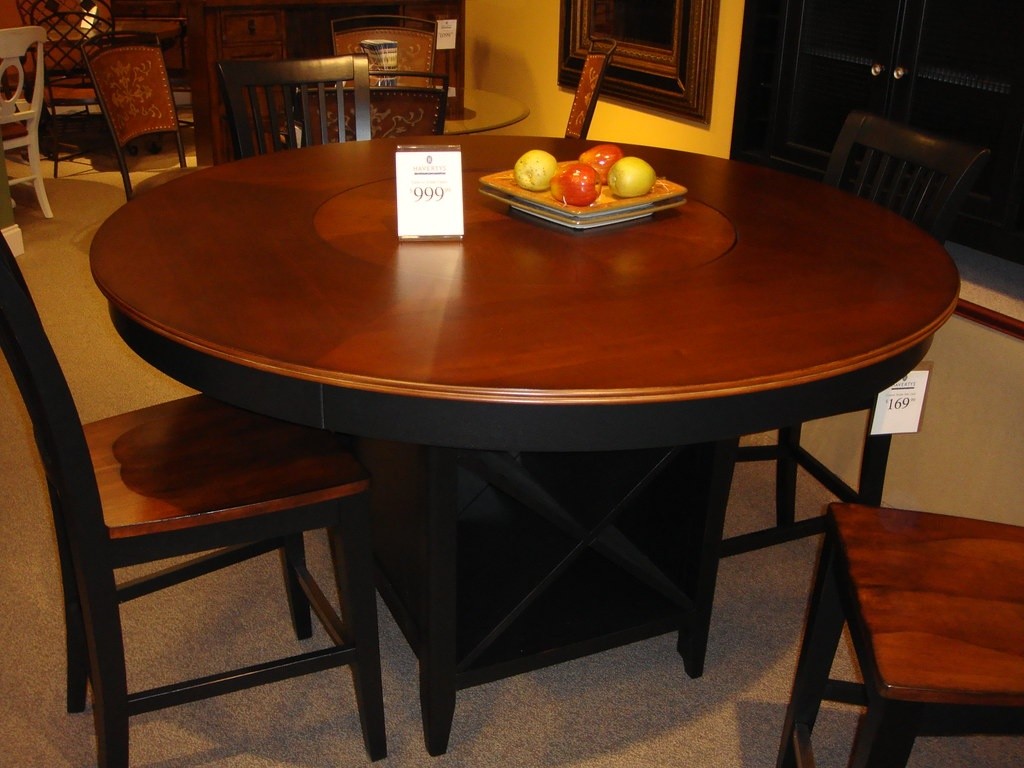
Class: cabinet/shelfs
187,0,468,166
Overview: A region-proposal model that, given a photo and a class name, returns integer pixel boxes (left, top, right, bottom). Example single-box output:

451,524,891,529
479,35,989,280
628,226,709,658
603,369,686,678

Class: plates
479,160,688,230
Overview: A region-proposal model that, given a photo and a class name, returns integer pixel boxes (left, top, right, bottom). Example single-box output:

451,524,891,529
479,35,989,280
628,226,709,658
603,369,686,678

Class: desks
19,19,188,130
90,135,961,758
343,74,530,135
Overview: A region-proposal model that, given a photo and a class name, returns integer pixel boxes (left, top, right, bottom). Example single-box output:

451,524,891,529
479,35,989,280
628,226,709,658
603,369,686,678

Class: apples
513,144,657,207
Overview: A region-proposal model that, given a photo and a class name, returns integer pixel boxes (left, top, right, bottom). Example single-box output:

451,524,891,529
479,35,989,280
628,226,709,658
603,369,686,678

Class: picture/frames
556,0,720,129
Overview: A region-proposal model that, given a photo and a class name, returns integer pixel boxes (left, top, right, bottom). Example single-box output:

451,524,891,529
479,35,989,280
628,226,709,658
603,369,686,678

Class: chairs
17,0,117,177
330,14,438,87
295,86,446,139
0,230,388,768
216,53,371,160
0,25,53,219
718,108,992,558
777,500,1024,767
